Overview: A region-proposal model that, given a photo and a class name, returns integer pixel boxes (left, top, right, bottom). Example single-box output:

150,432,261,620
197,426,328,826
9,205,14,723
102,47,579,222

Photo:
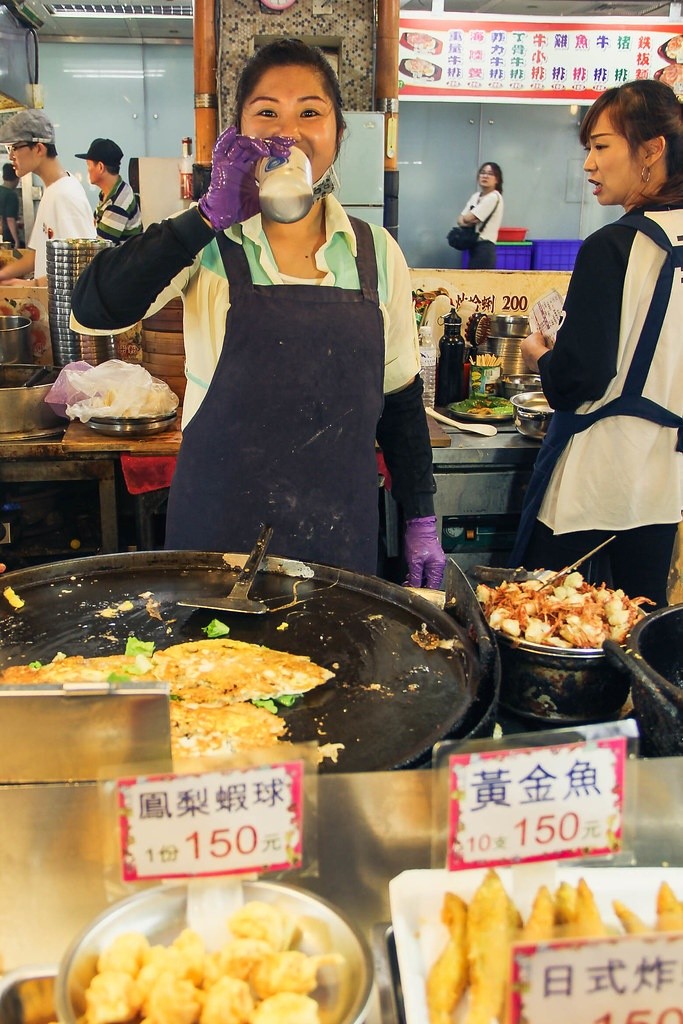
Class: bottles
435,308,469,407
418,326,437,410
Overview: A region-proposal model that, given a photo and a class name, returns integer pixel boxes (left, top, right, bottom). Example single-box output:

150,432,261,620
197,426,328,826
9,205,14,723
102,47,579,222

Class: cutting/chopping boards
61,416,451,453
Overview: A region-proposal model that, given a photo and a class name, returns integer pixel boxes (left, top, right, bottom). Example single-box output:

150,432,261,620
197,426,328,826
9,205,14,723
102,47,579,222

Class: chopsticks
469,354,502,366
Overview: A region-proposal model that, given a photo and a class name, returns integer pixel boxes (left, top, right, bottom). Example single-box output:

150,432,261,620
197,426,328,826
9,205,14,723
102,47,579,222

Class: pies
0,638,335,772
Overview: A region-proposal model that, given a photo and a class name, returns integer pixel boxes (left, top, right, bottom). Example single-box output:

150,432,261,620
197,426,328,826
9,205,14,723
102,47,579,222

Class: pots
0,548,503,787
0,363,65,433
491,609,646,728
602,601,683,758
509,391,554,440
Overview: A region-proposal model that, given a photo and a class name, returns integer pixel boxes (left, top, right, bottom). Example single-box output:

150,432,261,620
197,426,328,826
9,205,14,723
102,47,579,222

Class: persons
0,164,20,249
0,109,97,286
68,38,446,591
458,162,504,269
506,81,683,612
74,137,143,246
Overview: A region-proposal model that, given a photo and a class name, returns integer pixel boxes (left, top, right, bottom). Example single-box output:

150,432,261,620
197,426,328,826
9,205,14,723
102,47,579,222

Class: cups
258,146,314,224
470,365,500,397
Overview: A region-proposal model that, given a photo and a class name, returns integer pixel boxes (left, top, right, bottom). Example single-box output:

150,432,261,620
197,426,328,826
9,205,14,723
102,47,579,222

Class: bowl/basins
501,374,542,399
494,315,531,337
46,238,144,367
497,227,528,241
57,878,377,1023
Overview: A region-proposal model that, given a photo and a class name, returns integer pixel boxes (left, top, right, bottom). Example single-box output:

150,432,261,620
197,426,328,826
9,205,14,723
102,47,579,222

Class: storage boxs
528,238,584,270
461,246,531,270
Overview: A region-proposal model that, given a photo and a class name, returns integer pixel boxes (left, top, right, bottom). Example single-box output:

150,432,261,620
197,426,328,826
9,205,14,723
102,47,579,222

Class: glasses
7,142,37,153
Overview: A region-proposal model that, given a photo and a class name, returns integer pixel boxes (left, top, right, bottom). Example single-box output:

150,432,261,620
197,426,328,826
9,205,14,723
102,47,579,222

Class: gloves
403,515,446,590
198,125,296,232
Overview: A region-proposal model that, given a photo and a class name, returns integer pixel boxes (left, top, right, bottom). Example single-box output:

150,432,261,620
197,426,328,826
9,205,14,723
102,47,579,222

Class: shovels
176,522,273,613
425,406,498,437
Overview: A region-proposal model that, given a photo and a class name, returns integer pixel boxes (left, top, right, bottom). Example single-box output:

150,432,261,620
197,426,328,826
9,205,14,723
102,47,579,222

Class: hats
74,139,124,166
0,109,55,144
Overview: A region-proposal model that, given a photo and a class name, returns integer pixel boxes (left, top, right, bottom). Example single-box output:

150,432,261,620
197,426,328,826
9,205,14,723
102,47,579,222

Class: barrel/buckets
0,315,34,364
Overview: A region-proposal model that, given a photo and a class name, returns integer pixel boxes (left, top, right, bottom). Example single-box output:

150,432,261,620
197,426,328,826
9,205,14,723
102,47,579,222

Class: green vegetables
28,618,302,713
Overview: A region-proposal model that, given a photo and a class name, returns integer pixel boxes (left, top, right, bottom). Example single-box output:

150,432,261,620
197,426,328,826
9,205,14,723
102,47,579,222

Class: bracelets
198,203,210,222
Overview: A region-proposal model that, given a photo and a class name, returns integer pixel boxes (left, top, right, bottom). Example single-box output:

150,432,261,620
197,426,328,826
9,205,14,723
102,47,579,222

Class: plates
402,59,439,78
661,34,683,66
446,401,513,423
388,865,682,1024
85,409,178,437
403,32,438,50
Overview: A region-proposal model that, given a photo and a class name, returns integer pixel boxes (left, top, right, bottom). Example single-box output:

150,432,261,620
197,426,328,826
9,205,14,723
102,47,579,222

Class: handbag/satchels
444,224,479,251
